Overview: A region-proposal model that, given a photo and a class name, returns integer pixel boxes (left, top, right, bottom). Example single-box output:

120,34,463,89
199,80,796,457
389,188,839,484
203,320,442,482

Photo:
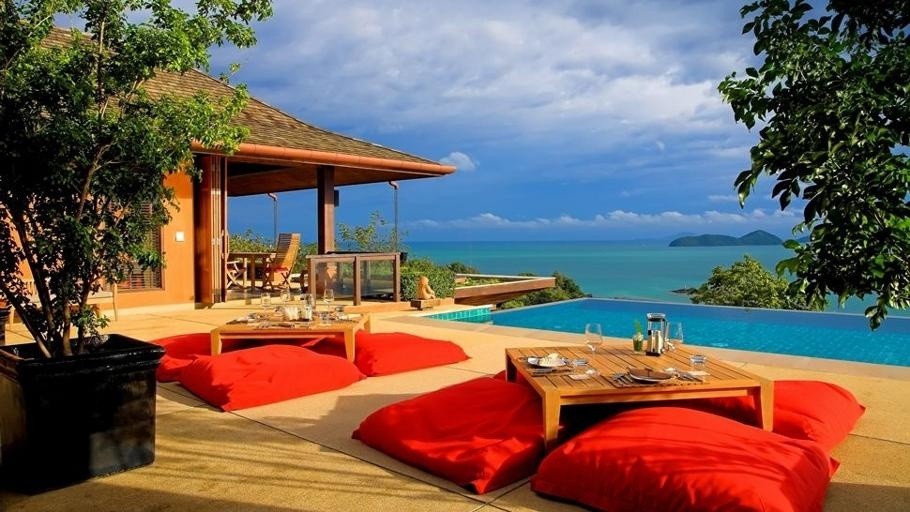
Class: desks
503,345,775,457
209,311,371,361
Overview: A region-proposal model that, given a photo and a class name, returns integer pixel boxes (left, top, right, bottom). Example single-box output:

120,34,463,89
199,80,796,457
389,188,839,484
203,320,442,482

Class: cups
632,332,643,352
260,293,271,321
280,290,290,307
690,355,707,371
646,312,668,356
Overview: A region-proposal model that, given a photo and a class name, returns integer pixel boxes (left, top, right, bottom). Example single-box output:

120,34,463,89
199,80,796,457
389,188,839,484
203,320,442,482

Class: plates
629,367,680,382
527,354,566,368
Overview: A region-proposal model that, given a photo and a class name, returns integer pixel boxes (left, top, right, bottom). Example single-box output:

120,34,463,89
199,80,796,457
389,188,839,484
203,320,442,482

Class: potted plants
0,0,274,494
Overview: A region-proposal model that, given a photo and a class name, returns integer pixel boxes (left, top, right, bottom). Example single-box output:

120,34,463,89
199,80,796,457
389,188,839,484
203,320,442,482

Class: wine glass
664,321,683,372
585,323,602,376
324,289,334,312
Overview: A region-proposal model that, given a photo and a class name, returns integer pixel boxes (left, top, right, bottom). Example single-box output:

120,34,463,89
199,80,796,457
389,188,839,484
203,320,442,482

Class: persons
417,275,436,301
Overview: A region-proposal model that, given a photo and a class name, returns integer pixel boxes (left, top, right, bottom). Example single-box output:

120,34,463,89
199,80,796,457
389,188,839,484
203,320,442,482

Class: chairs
226,231,301,295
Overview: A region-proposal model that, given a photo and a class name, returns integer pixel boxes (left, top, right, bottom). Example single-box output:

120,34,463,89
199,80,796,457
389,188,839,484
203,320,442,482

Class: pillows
348,369,866,512
147,330,471,414
